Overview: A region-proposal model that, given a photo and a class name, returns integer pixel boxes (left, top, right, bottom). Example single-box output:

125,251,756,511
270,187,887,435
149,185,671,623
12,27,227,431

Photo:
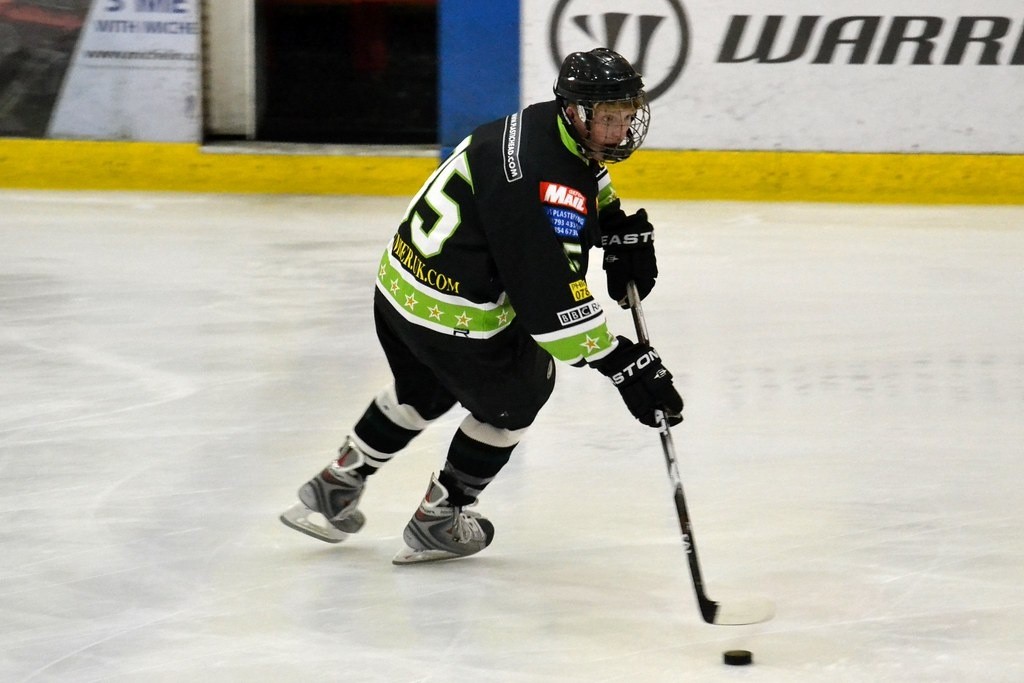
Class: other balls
724,649,752,665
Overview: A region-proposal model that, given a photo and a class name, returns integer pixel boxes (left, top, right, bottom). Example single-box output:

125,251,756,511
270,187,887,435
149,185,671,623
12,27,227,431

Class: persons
280,47,684,565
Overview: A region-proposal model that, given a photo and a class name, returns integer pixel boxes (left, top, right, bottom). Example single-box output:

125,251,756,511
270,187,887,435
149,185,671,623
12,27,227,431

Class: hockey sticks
626,278,776,625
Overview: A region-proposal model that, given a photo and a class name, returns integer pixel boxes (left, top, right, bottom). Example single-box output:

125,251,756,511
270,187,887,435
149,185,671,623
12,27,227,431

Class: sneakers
281,436,365,544
392,470,494,565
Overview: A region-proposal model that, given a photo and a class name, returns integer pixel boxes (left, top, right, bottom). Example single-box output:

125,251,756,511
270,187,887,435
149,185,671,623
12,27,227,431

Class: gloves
595,209,658,310
571,335,684,428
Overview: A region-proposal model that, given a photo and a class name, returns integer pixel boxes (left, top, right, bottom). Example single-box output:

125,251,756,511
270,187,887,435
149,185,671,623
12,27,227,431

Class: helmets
552,48,651,164
